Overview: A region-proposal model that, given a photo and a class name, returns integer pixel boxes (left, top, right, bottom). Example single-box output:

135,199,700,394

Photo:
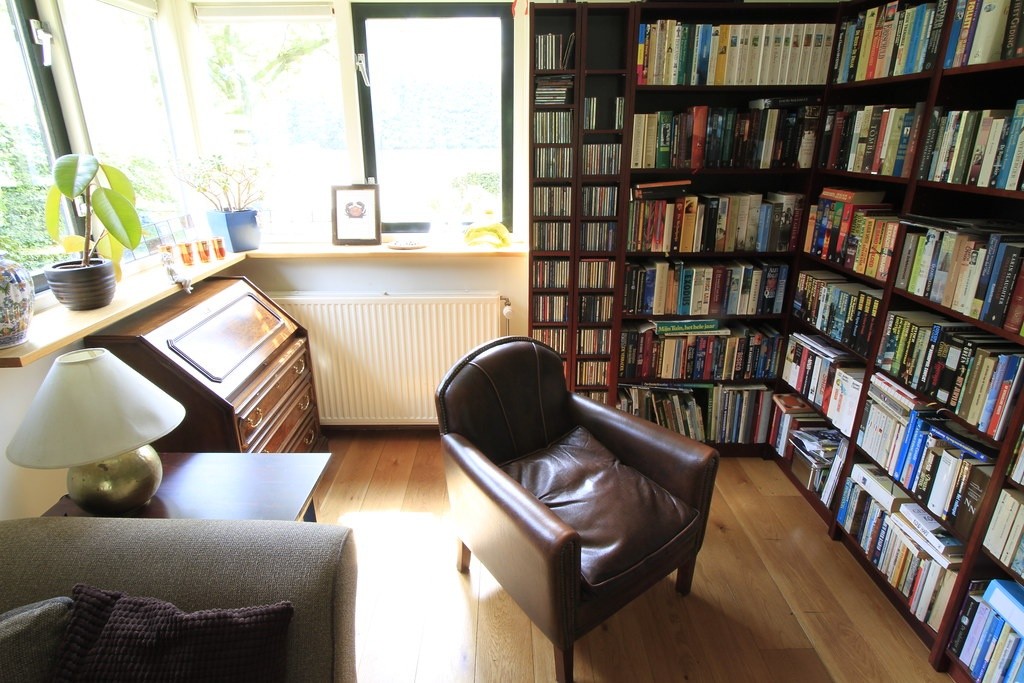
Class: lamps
6,348,186,517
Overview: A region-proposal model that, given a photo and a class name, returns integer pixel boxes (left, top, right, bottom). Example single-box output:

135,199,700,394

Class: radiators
264,291,513,430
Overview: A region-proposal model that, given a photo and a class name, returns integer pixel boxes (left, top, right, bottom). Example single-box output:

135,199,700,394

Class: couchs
0,520,356,683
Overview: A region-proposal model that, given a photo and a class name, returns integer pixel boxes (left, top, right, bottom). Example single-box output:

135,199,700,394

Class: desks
38,453,334,522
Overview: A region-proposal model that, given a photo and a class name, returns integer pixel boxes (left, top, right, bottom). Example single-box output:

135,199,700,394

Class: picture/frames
331,185,382,247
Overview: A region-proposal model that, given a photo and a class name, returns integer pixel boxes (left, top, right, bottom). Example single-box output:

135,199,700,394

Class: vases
0,260,35,349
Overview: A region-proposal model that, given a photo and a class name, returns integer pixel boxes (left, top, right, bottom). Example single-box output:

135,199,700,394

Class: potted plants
44,153,143,312
171,151,262,253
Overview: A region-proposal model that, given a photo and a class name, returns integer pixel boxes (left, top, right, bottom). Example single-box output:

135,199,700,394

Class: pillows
0,597,73,683
42,584,295,683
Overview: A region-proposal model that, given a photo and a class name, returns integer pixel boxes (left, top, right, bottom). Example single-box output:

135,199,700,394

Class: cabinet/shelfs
85,276,328,453
526,0,1024,683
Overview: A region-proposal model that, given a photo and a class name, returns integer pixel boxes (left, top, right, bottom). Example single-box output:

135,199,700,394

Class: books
532,0,1024,683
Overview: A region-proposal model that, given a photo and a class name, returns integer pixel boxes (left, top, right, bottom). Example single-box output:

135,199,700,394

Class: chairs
435,335,720,683
